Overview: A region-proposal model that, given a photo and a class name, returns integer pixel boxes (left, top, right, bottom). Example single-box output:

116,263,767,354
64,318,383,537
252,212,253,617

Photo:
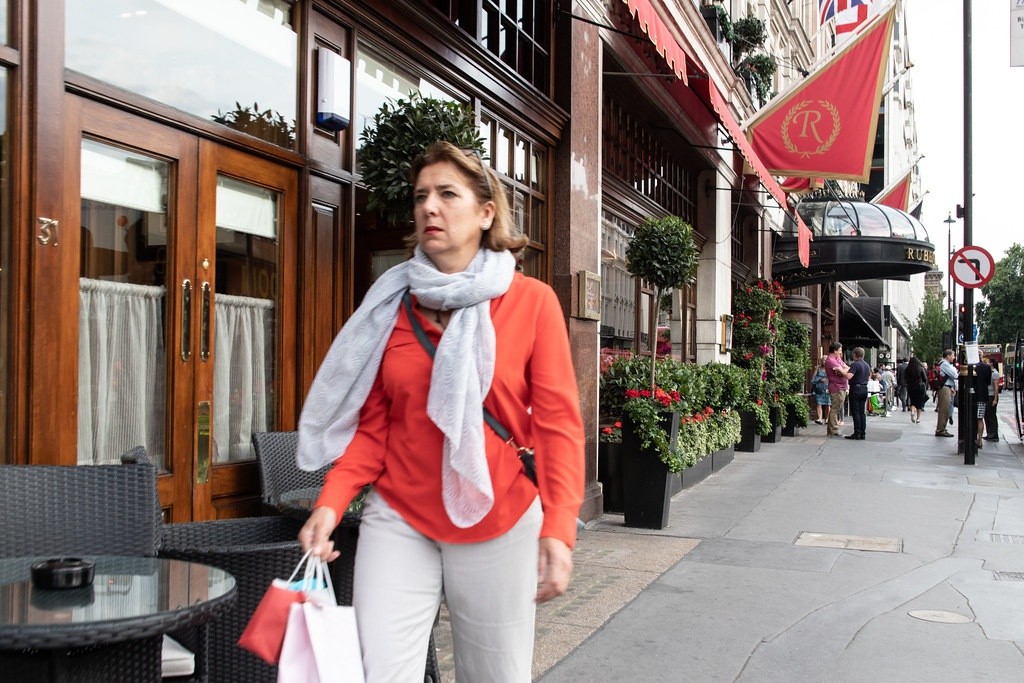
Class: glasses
460,147,495,202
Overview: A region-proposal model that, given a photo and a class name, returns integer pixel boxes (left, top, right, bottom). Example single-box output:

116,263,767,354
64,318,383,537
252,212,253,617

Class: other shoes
978,444,983,448
902,409,906,412
916,420,920,424
911,413,915,423
949,416,953,425
983,436,999,442
935,429,954,437
908,407,911,411
844,433,865,439
815,420,823,425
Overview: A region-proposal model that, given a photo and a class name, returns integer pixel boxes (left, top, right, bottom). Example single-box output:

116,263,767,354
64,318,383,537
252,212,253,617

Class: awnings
621,0,789,214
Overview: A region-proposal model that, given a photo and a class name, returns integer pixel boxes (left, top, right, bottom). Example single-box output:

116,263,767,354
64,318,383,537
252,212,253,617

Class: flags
875,171,911,213
779,176,824,193
742,0,897,185
816,0,870,34
909,200,923,220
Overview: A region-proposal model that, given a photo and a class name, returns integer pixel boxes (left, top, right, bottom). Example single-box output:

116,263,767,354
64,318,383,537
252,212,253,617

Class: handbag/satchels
237,546,365,683
812,381,823,395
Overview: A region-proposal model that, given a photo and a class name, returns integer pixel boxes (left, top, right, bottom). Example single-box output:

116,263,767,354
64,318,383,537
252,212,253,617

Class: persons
297,141,586,683
811,341,999,449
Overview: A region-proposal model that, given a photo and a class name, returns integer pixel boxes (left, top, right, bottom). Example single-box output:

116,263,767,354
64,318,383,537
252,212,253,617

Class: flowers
600,213,789,476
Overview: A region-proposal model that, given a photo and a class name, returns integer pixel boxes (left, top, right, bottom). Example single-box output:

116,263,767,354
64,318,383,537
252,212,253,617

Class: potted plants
782,315,812,437
622,216,700,529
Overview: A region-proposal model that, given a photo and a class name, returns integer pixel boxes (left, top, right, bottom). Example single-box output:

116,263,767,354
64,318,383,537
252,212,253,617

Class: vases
600,403,797,514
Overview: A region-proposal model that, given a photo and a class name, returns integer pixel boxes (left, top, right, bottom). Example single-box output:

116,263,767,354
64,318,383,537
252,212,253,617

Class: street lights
943,211,957,368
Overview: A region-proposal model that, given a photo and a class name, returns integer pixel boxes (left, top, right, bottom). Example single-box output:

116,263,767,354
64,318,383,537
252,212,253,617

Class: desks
0,556,239,683
275,486,440,683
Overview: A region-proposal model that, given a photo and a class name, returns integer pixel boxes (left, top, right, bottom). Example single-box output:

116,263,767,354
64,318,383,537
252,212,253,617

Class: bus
1005,343,1024,390
957,343,1005,393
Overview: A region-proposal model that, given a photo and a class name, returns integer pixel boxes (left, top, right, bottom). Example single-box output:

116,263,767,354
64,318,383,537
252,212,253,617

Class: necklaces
435,310,446,329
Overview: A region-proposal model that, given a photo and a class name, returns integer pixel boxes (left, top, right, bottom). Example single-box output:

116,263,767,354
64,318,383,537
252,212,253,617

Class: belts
851,383,867,387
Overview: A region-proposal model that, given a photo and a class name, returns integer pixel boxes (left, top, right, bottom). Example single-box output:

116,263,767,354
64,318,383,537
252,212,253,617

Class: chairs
0,432,333,683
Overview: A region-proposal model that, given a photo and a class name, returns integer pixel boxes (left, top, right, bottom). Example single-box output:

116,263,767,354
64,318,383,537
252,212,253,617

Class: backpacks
928,361,950,392
